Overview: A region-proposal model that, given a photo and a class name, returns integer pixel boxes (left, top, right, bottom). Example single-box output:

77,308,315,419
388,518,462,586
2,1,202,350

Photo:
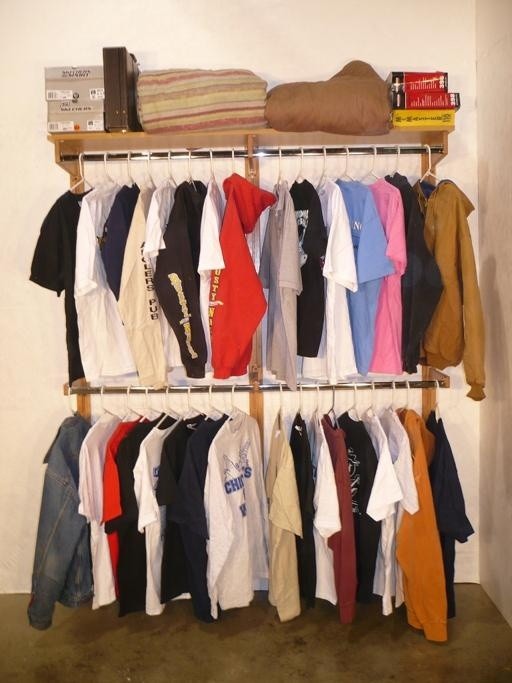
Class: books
386,71,460,133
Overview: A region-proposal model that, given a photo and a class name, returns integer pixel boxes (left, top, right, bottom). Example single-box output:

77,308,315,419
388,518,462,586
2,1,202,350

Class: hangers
62,148,444,195
64,379,445,433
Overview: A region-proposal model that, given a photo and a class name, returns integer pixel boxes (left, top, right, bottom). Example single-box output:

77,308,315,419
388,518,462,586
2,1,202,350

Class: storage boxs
42,66,108,137
